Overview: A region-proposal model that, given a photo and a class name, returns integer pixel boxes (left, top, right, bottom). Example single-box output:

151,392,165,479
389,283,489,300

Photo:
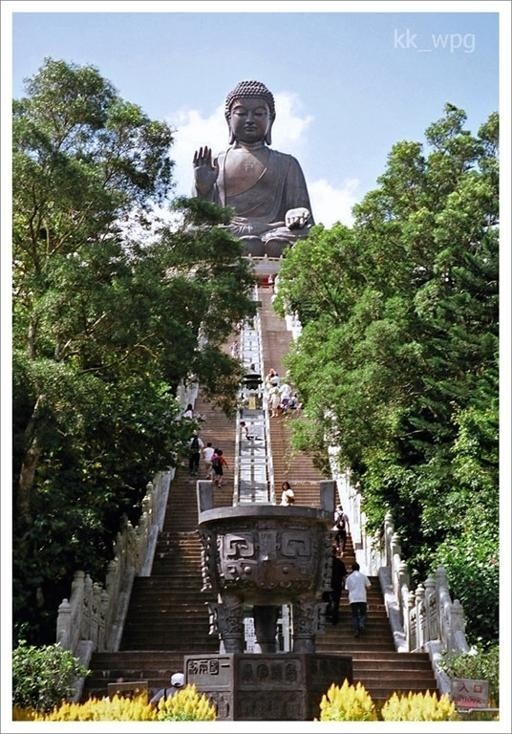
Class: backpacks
212,458,221,468
336,513,345,529
190,437,200,449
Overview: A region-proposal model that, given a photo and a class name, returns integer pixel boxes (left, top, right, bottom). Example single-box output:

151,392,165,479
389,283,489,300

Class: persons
173,364,310,488
343,560,371,638
187,79,317,258
321,545,346,628
332,505,351,557
277,480,296,507
148,672,186,709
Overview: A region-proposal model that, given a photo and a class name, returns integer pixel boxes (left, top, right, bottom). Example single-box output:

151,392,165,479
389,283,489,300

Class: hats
171,673,185,686
